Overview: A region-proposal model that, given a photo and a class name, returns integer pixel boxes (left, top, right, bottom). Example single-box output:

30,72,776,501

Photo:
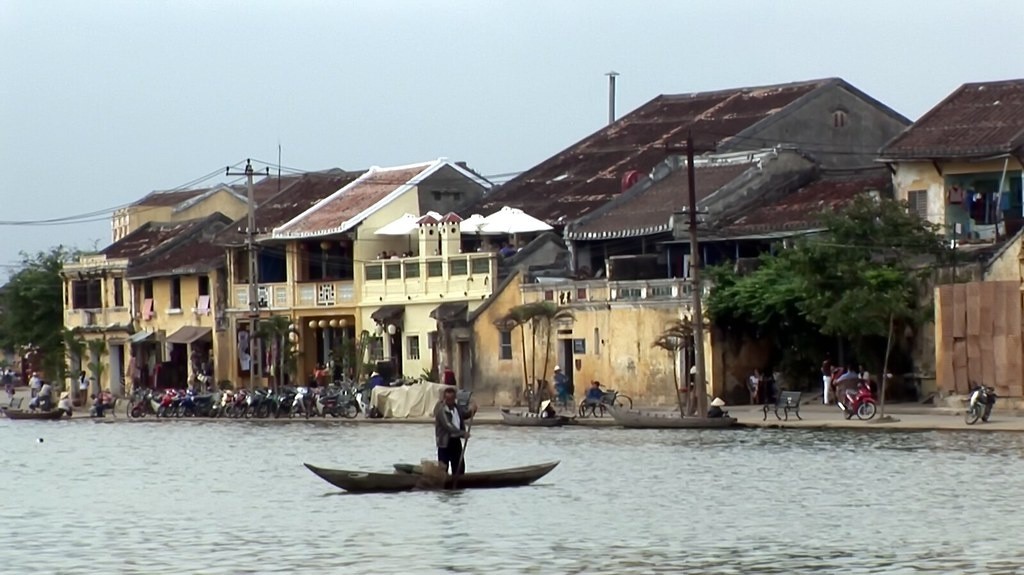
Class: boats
602,403,738,429
499,407,576,425
303,460,563,493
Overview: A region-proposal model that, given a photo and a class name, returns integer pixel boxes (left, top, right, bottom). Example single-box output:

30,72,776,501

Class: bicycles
523,383,552,404
578,380,632,416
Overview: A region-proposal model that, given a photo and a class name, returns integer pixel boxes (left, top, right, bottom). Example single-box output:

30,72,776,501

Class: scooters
963,380,999,425
125,380,361,419
836,369,877,421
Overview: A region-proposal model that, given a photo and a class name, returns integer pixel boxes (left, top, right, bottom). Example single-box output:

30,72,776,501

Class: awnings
162,325,212,345
120,329,156,343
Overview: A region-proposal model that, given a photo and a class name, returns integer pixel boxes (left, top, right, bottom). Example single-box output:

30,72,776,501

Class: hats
60,391,70,399
554,365,561,371
690,365,696,374
541,400,551,411
371,371,379,378
710,397,725,406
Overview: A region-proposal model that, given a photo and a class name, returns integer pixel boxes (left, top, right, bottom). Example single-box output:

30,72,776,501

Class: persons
379,240,525,258
820,360,871,404
370,371,384,390
77,370,90,406
90,394,101,417
707,397,726,419
433,388,478,475
57,391,73,416
28,372,52,411
749,368,763,403
442,366,457,385
539,399,556,418
554,365,569,414
586,381,605,417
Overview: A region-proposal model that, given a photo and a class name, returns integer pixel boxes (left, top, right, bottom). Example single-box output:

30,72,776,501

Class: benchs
0,396,25,412
580,391,618,417
763,390,803,422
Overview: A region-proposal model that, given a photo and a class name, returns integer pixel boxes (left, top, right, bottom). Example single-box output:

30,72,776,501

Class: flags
235,329,251,377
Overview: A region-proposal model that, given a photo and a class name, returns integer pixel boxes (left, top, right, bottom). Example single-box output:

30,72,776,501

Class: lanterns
309,319,348,328
388,324,396,344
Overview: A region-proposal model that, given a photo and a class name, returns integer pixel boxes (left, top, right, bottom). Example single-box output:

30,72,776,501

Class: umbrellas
374,206,554,251
